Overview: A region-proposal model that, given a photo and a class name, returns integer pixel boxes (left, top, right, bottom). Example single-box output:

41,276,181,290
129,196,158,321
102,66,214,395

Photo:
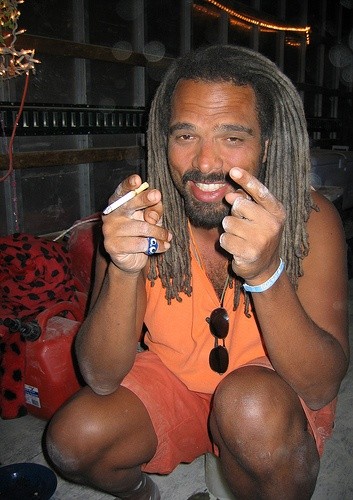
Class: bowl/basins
0,463,57,500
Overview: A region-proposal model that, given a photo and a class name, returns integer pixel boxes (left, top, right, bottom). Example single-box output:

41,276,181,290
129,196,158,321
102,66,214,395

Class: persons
46,42,349,500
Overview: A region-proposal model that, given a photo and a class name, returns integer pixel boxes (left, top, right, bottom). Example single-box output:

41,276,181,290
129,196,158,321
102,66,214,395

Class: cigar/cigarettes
102,181,149,215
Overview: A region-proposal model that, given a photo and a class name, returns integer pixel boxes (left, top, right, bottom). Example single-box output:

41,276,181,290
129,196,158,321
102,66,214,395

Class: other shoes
114,473,161,500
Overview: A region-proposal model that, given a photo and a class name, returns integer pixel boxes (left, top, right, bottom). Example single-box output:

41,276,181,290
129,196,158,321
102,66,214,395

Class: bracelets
243,257,284,292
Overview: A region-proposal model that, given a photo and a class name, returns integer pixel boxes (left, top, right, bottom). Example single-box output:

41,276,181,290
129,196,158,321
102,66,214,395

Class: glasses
206,282,229,375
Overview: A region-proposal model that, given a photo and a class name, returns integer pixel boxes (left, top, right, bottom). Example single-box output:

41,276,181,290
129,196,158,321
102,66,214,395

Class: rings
145,237,158,255
